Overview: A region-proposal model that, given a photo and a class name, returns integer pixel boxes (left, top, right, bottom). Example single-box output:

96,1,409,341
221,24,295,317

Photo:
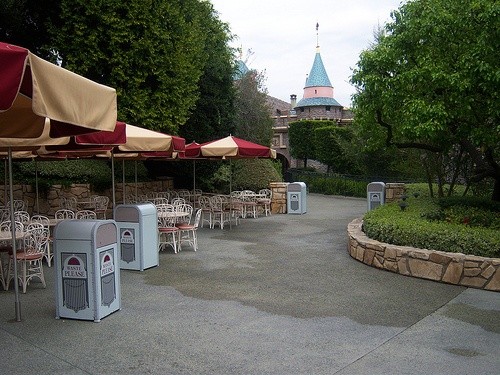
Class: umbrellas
2,120,282,218
1,41,120,323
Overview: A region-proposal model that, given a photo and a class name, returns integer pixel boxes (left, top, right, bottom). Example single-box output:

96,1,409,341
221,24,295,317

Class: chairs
0,189,273,293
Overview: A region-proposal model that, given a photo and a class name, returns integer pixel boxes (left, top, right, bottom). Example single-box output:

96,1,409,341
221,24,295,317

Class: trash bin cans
288,181,307,216
54,219,121,322
366,182,385,211
114,203,160,272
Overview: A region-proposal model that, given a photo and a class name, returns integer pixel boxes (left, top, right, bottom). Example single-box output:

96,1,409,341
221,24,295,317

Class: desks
240,192,265,216
26,221,73,248
182,192,202,205
74,199,94,216
213,199,227,229
0,228,33,265
155,205,189,244
148,194,165,202
